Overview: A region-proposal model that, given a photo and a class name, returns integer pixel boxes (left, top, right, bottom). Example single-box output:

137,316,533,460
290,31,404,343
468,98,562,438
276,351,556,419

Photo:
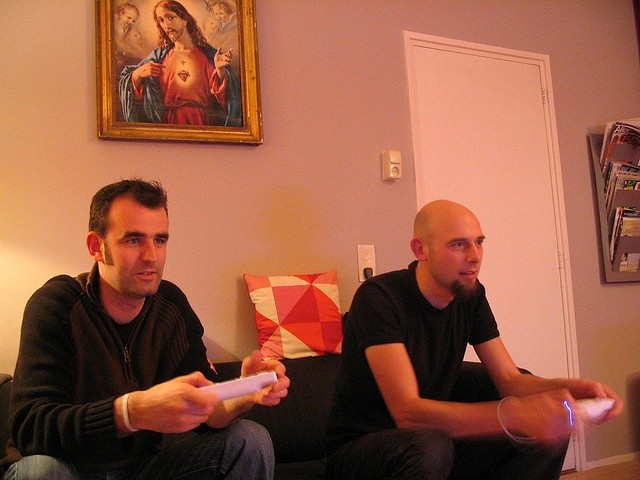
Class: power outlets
358,244,377,283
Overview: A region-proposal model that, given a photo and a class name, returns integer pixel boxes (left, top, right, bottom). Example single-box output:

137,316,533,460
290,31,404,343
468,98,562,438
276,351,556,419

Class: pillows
243,270,345,362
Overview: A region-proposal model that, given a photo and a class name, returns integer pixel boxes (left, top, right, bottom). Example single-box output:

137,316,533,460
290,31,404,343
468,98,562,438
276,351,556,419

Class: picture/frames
93,1,263,146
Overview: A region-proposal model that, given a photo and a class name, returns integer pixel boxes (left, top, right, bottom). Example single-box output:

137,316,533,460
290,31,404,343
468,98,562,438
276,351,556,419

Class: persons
210,0,240,73
324,199,625,480
202,16,222,43
118,0,243,128
114,3,144,61
126,25,144,48
2,178,291,480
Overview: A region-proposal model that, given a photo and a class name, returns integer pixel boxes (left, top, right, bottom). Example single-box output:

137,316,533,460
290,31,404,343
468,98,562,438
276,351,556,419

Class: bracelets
122,393,138,434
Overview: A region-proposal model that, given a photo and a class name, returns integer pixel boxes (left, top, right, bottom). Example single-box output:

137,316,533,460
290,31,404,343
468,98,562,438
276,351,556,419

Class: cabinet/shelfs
587,117,640,283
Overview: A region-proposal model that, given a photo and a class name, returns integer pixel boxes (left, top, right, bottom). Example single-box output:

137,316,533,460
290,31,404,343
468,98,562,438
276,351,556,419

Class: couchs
0,352,533,480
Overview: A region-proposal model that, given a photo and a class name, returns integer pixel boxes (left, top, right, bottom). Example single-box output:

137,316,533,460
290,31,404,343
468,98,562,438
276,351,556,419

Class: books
600,118,640,273
